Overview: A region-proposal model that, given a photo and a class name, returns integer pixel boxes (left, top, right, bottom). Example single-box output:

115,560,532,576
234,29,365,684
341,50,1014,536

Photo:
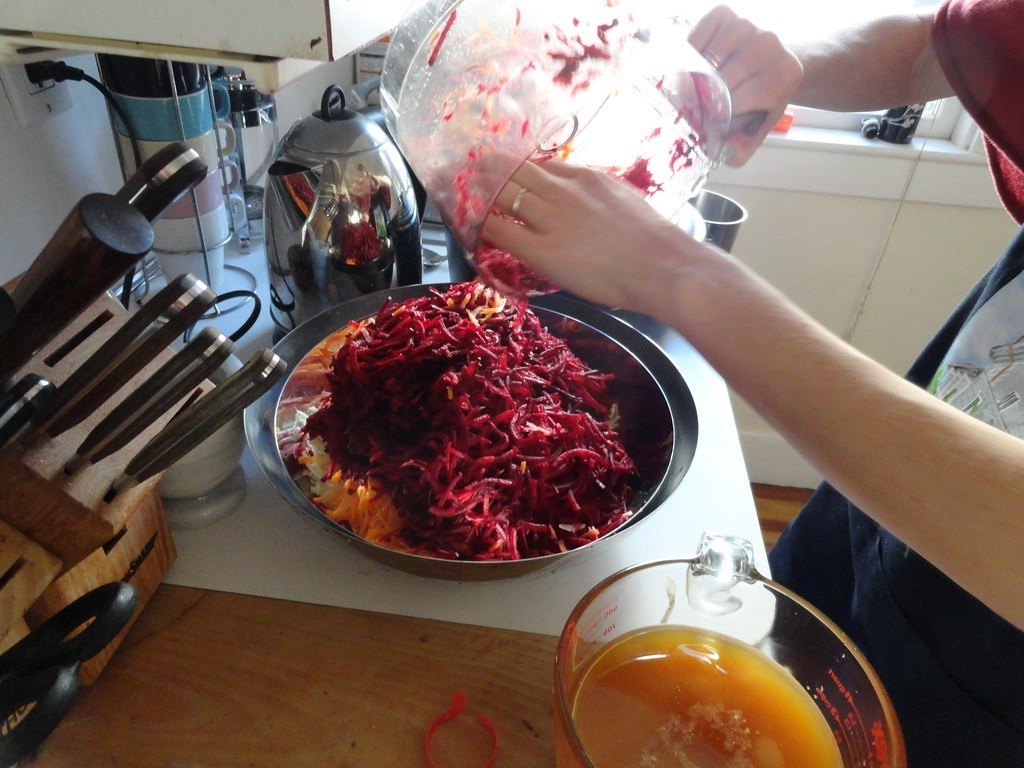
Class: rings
702,49,720,70
512,188,527,213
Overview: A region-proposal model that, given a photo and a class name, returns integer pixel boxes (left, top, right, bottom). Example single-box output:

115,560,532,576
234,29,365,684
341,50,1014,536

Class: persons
436,0,1024,768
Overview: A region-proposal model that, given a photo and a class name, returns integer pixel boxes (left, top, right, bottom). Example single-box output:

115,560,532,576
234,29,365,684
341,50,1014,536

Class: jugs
211,65,280,220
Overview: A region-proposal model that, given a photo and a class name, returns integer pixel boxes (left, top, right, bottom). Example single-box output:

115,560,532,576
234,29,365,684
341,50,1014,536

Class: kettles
264,84,425,347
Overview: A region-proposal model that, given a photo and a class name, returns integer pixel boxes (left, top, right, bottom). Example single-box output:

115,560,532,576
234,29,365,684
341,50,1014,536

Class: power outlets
0,60,75,128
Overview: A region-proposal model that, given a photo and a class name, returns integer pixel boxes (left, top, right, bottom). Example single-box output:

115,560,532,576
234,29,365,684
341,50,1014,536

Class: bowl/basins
244,282,699,583
555,532,909,768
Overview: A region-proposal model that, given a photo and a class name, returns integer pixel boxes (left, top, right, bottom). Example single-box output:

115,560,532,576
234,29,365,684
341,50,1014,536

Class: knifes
0,144,287,497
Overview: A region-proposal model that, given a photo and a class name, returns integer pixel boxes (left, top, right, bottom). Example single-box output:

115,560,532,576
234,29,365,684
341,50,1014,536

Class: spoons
422,248,442,266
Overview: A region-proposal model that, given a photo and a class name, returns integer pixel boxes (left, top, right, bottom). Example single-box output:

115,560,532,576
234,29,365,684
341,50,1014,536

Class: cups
689,189,748,254
97,54,248,296
877,102,926,144
375,0,772,300
